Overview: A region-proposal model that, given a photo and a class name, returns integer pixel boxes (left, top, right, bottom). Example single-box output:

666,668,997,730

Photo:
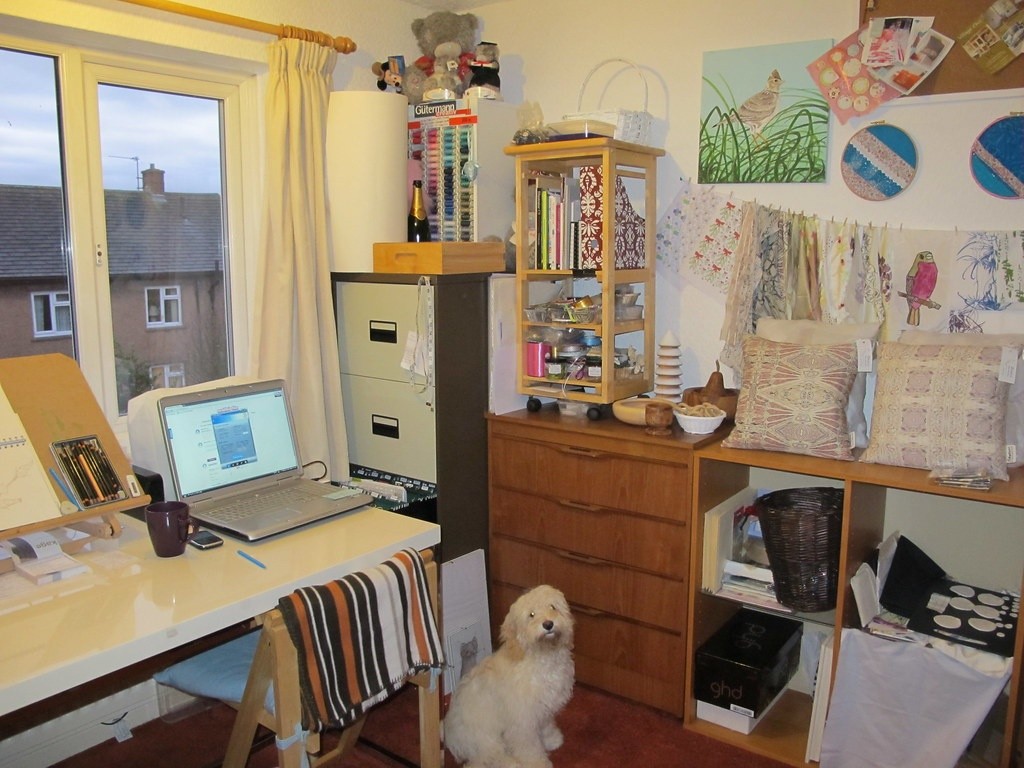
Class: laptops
159,378,374,542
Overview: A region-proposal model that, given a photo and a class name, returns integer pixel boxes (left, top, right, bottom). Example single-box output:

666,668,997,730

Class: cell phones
187,531,224,549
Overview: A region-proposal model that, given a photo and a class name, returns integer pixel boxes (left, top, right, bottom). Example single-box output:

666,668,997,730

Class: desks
0,505,442,768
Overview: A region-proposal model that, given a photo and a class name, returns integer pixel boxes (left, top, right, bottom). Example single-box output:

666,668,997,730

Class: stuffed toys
372,61,403,94
401,11,500,106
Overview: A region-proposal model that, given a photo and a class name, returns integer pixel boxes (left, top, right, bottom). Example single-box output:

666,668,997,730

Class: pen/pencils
238,550,266,569
59,440,122,507
49,467,81,511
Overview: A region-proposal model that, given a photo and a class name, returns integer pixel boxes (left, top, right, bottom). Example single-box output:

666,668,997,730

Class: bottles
544,356,568,381
407,180,432,242
583,358,602,384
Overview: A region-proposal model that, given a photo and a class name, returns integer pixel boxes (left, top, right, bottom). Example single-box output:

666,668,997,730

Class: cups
526,341,553,378
144,501,199,558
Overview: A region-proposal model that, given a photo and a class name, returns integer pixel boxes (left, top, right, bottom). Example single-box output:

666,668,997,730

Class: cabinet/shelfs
484,400,1024,768
504,137,666,405
329,272,494,640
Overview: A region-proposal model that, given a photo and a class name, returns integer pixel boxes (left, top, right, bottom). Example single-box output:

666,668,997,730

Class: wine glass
645,402,674,436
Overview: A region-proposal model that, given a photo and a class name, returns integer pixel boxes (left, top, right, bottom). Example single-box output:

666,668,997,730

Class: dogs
438,584,574,768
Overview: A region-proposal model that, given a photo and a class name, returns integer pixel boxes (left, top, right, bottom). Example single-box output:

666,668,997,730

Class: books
722,559,778,602
525,174,581,270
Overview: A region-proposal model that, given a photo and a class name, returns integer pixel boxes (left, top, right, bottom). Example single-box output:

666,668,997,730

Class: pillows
720,316,1024,482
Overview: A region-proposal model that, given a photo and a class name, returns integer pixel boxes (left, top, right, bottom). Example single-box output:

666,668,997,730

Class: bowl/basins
556,398,593,416
524,287,643,325
674,407,726,434
545,119,618,142
612,397,674,425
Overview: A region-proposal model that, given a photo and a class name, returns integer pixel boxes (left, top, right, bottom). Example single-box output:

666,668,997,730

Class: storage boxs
527,341,552,378
523,305,644,325
373,241,506,274
545,119,617,142
693,607,804,735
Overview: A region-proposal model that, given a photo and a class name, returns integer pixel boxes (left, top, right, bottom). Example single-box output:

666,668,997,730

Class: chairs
152,548,441,768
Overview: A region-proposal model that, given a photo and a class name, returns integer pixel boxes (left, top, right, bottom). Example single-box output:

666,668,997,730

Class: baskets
562,57,660,146
755,488,844,612
